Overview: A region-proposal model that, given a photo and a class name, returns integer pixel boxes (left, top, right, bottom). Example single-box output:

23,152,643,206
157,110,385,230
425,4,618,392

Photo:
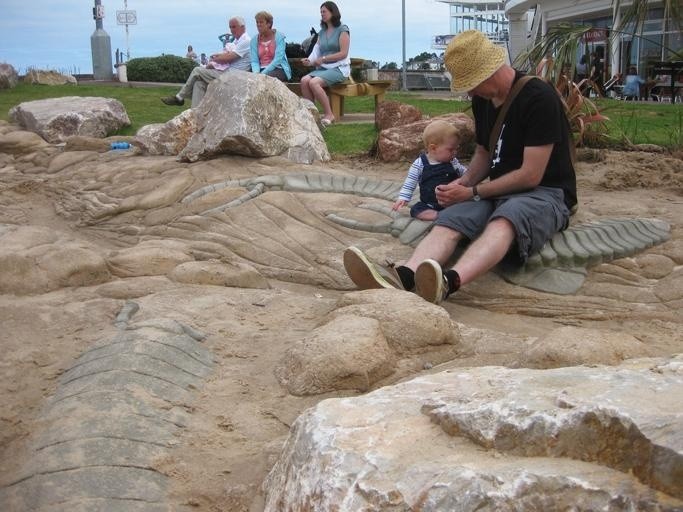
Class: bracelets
321,54,326,65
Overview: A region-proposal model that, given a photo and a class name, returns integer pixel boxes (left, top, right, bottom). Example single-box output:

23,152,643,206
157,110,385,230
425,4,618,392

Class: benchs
281,78,396,123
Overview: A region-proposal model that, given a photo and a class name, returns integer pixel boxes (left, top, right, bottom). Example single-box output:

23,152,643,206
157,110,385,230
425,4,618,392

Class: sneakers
415,259,447,307
344,245,406,291
159,94,185,107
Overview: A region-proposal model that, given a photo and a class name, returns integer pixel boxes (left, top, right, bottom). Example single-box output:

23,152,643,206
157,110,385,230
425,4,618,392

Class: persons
392,120,468,222
344,28,579,306
161,15,251,109
250,9,293,92
216,32,235,49
200,52,209,65
185,45,197,60
298,1,352,129
577,51,670,101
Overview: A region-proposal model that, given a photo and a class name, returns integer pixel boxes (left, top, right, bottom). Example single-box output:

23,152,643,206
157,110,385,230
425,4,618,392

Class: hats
445,30,508,94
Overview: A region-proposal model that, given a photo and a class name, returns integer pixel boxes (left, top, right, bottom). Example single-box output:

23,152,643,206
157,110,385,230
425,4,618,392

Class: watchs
472,186,481,202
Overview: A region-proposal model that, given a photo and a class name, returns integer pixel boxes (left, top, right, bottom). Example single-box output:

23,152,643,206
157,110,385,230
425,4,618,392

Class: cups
367,69,379,81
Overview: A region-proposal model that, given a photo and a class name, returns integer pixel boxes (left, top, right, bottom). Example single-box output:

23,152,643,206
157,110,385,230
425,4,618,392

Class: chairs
607,74,682,106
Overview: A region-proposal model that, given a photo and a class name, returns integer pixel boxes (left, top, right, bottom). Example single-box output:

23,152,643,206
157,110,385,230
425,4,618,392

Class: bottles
110,142,132,149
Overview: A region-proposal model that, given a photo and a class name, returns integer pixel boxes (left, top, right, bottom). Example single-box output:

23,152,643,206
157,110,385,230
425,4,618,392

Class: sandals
322,114,335,126
